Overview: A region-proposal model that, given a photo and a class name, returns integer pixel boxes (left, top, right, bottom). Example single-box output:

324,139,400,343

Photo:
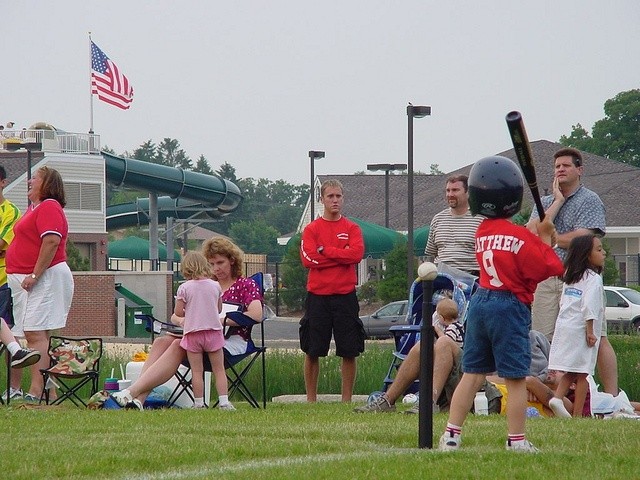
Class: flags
91,40,133,112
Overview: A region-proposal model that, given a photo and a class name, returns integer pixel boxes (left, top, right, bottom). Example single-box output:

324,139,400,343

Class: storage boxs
126,363,211,408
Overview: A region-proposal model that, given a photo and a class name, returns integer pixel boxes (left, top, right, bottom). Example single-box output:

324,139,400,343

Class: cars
602,285,640,329
359,300,408,339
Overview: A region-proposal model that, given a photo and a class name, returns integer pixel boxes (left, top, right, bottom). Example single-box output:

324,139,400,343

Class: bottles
116,379,132,391
104,378,119,398
473,388,488,418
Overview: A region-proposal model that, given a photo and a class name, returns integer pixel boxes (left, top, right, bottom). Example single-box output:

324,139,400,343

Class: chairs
134,272,266,409
0,287,15,405
39,336,102,407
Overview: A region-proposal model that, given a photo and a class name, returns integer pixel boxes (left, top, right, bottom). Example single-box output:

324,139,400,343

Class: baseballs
418,262,438,281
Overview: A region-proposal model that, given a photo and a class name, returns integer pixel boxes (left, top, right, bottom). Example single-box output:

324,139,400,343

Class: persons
0,165,23,330
525,149,619,397
436,299,465,409
110,236,264,411
4,165,74,402
424,175,488,277
174,253,236,411
0,316,42,369
438,156,566,456
353,335,573,428
527,330,552,377
299,179,368,403
548,235,607,420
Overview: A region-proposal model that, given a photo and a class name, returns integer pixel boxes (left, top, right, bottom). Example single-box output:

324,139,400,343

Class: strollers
367,262,479,409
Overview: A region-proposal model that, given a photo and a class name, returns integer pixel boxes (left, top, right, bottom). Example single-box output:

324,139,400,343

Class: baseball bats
505,111,558,249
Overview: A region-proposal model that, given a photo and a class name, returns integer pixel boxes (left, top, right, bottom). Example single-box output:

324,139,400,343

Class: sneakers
2,387,23,400
505,440,541,454
25,393,38,401
438,431,461,451
354,393,397,413
191,402,206,409
11,349,41,369
219,400,237,410
404,392,440,414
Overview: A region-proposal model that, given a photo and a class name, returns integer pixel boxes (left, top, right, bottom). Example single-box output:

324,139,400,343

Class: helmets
467,155,524,218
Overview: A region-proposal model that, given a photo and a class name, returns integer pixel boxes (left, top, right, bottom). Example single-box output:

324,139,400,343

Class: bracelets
318,246,325,254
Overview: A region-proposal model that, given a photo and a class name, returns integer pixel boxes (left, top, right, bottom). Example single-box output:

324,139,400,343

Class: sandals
111,389,133,407
125,399,144,411
